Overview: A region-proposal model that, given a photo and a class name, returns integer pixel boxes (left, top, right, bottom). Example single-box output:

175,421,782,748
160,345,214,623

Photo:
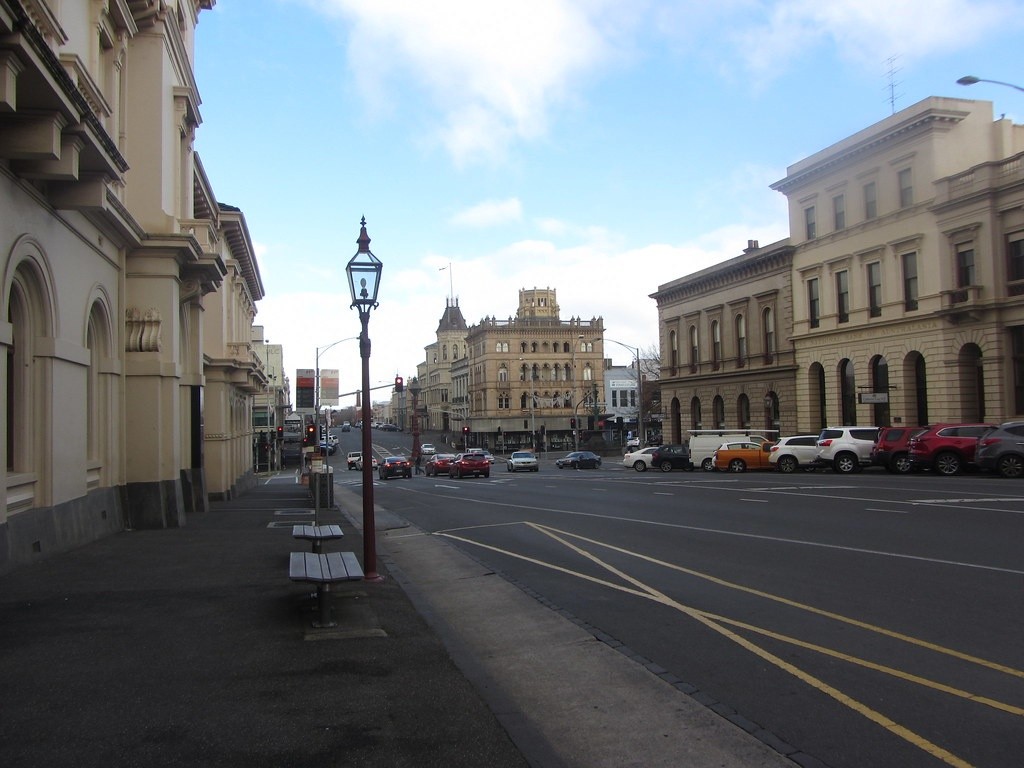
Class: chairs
741,444,746,449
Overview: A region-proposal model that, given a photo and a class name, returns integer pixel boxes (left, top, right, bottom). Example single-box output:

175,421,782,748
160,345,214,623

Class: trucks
689,434,769,473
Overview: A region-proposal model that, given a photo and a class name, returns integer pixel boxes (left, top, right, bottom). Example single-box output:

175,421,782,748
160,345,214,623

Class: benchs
289,551,365,630
292,523,344,554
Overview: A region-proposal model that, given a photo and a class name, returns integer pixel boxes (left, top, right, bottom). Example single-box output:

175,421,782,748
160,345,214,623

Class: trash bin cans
649,442,659,447
309,464,334,507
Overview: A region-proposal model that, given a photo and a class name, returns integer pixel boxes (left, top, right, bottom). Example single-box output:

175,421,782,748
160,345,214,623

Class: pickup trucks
505,451,539,472
711,441,774,472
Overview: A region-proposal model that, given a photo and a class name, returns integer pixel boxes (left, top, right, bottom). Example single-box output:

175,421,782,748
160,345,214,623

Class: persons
415,453,426,474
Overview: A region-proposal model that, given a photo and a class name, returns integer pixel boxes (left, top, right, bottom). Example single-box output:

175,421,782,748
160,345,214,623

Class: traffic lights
541,425,545,434
302,437,308,447
277,426,284,437
462,426,468,435
521,392,526,408
524,419,528,428
307,426,316,442
617,417,623,428
571,418,575,429
395,377,403,393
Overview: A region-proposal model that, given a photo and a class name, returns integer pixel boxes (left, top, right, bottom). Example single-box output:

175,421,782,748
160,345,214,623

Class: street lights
571,335,600,450
345,215,383,580
314,336,360,448
593,337,645,448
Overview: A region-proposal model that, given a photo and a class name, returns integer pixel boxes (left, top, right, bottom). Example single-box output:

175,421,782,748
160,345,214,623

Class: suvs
814,426,879,473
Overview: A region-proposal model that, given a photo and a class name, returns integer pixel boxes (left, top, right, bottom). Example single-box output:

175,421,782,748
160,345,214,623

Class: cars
908,422,996,475
319,420,402,455
974,421,1024,479
424,454,455,476
421,444,437,455
447,452,490,479
348,451,378,470
712,442,763,471
871,426,923,474
467,447,495,463
769,435,820,473
651,444,689,472
623,447,677,472
555,451,602,470
378,457,412,479
